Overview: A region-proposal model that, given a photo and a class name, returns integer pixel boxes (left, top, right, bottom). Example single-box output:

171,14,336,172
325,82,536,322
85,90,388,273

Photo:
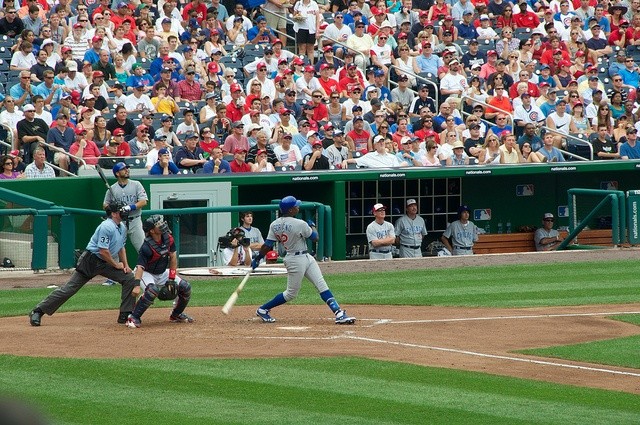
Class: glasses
353,92,360,94
400,49,408,51
620,118,627,121
46,44,53,46
146,117,153,119
65,51,72,54
505,10,512,12
445,35,451,36
167,61,173,64
351,4,358,6
376,115,383,118
350,68,356,70
23,49,31,53
43,30,51,32
204,131,211,133
488,139,496,142
630,132,637,134
47,77,54,79
571,33,578,35
227,76,233,78
8,12,16,13
422,90,429,92
522,75,529,76
29,0,37,2
447,119,455,121
165,119,172,122
572,96,579,98
327,129,334,131
533,33,540,36
314,95,322,98
399,115,407,118
97,18,104,19
427,28,433,29
382,126,388,129
303,125,310,127
280,62,287,65
79,20,88,22
214,53,221,55
337,17,343,18
505,32,512,33
6,100,14,102
601,109,608,111
237,127,243,129
404,143,411,145
253,84,261,86
377,208,385,211
431,146,438,149
445,57,452,58
525,44,532,46
140,23,146,26
21,77,31,79
450,135,456,137
313,145,322,148
7,0,14,3
455,147,463,149
473,127,480,130
592,80,598,82
474,68,481,71
266,52,273,55
555,53,561,55
160,138,166,141
545,219,554,222
524,146,532,148
615,8,622,10
189,65,195,67
424,46,430,49
188,72,195,75
285,137,292,140
355,109,361,112
511,56,518,59
116,134,125,137
474,110,483,112
496,78,502,80
380,37,387,40
615,79,622,82
355,14,362,16
190,42,197,43
422,37,428,38
498,118,506,120
309,107,314,110
561,5,569,7
617,55,626,57
332,97,340,99
288,94,295,96
371,91,377,93
472,80,479,82
621,25,628,28
377,209,385,211
423,111,430,113
294,64,304,66
192,15,198,18
4,163,13,165
213,12,218,14
626,60,634,63
260,69,267,71
64,97,72,100
358,26,365,28
401,79,408,82
469,120,477,122
80,131,87,136
27,110,35,112
137,89,145,91
424,120,432,122
421,16,428,19
80,9,87,11
105,13,111,15
283,113,290,116
497,88,505,90
327,50,334,53
401,38,407,40
590,71,598,73
549,31,557,33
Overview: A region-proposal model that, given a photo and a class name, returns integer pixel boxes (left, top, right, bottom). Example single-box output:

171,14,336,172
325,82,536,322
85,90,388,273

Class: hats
412,136,421,142
443,30,451,34
608,3,627,15
463,10,472,15
371,98,383,106
122,19,131,24
61,46,72,53
160,114,172,122
407,199,417,206
312,140,322,147
324,122,334,130
545,22,554,29
138,4,149,11
444,16,452,21
366,65,378,74
55,113,67,120
495,59,506,67
294,58,305,65
93,70,105,77
257,62,266,69
425,130,435,137
249,123,263,132
471,64,481,71
22,104,35,116
183,108,194,115
59,92,72,101
189,18,201,28
208,62,218,72
530,28,544,38
10,149,23,159
303,105,316,109
380,21,392,28
320,64,330,70
544,9,553,16
117,2,127,8
305,65,316,72
234,16,243,22
40,39,57,52
275,76,286,82
520,60,533,66
353,116,363,124
128,4,135,12
260,92,270,99
205,93,217,99
575,38,587,44
519,1,527,6
573,101,582,108
558,60,572,69
398,74,407,80
72,23,83,28
556,99,566,105
185,67,195,73
568,81,578,87
443,49,455,57
422,41,431,47
299,120,310,126
347,63,357,70
553,49,562,55
210,29,219,36
521,93,530,99
335,12,344,17
469,75,480,84
418,84,429,90
278,59,287,65
366,86,381,101
620,19,628,26
137,125,149,131
626,127,638,134
401,20,411,26
141,130,149,133
161,66,172,73
81,108,94,114
372,203,386,212
154,133,167,141
592,89,602,95
266,251,277,259
279,108,290,115
273,39,282,45
480,14,488,21
547,88,557,94
468,39,479,45
576,51,585,57
284,69,294,75
589,16,596,22
283,133,292,139
351,86,361,91
398,33,407,39
74,127,88,135
419,11,428,16
185,132,198,140
487,50,497,56
211,49,222,54
230,83,241,92
92,36,103,42
571,16,581,22
452,141,465,149
424,21,434,28
113,128,125,136
375,10,384,16
344,52,354,57
265,46,272,52
584,63,592,68
617,114,627,120
307,131,318,140
162,18,171,23
587,66,597,73
233,121,244,128
352,105,362,113
71,91,81,105
84,94,95,101
234,148,247,154
588,76,598,81
285,89,295,95
374,68,384,76
353,11,362,16
324,46,333,52
471,103,483,110
66,60,77,71
332,130,345,138
378,32,388,37
355,20,365,28
250,110,261,117
106,139,119,147
164,56,174,61
257,150,267,155
142,111,154,117
374,135,385,144
545,213,554,218
540,64,550,70
591,24,600,30
183,46,193,52
449,60,460,65
256,16,266,22
158,149,169,160
540,82,550,88
132,81,144,88
83,61,91,68
108,84,124,92
401,136,412,145
469,124,481,130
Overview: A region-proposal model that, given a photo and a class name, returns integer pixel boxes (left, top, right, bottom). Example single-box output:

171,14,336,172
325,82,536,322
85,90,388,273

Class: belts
127,216,140,220
372,250,389,253
401,244,419,249
295,251,309,255
456,247,471,250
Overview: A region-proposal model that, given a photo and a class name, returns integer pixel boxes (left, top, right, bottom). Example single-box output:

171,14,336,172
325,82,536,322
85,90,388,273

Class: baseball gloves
158,280,179,301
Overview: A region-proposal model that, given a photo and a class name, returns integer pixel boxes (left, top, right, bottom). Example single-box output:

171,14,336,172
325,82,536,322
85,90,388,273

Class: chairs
1,30,38,103
447,26,532,62
565,132,589,147
85,57,209,138
587,44,640,101
589,131,613,147
221,38,265,92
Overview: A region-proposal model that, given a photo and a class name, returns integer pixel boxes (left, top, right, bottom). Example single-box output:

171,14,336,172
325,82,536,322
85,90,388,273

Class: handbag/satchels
576,145,590,160
417,72,437,85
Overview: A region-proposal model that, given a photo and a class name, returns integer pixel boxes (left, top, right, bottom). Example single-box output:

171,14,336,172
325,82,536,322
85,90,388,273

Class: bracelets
133,278,141,286
169,268,176,280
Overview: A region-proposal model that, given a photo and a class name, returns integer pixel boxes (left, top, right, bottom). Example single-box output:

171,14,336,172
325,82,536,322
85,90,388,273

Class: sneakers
541,157,547,162
335,310,356,324
551,156,557,162
102,278,119,286
118,312,132,323
30,309,41,325
170,312,193,322
126,315,141,329
256,307,276,323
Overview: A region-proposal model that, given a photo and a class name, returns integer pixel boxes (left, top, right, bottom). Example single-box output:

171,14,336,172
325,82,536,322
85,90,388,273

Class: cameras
540,38,549,41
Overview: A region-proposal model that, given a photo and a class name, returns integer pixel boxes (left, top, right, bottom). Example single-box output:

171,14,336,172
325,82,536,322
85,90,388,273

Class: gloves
251,260,259,269
308,220,316,227
122,205,137,212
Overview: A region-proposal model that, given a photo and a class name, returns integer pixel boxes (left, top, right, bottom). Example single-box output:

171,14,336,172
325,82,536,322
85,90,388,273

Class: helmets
105,201,132,223
144,215,173,237
113,162,130,172
457,204,471,218
280,196,301,214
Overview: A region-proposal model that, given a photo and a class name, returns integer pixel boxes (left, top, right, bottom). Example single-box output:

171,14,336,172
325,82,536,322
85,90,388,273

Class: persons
216,228,252,266
440,204,480,256
29,200,135,327
354,0,637,169
534,213,562,252
366,203,395,259
1,0,357,175
103,162,146,255
395,198,428,258
266,251,279,264
238,212,265,265
253,195,356,324
126,213,195,327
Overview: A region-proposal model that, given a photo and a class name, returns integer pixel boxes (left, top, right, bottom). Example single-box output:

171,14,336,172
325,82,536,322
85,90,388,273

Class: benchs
470,228,630,255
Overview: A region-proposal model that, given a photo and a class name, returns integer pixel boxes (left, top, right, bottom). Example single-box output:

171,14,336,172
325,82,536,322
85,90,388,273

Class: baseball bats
221,265,253,315
95,164,119,203
